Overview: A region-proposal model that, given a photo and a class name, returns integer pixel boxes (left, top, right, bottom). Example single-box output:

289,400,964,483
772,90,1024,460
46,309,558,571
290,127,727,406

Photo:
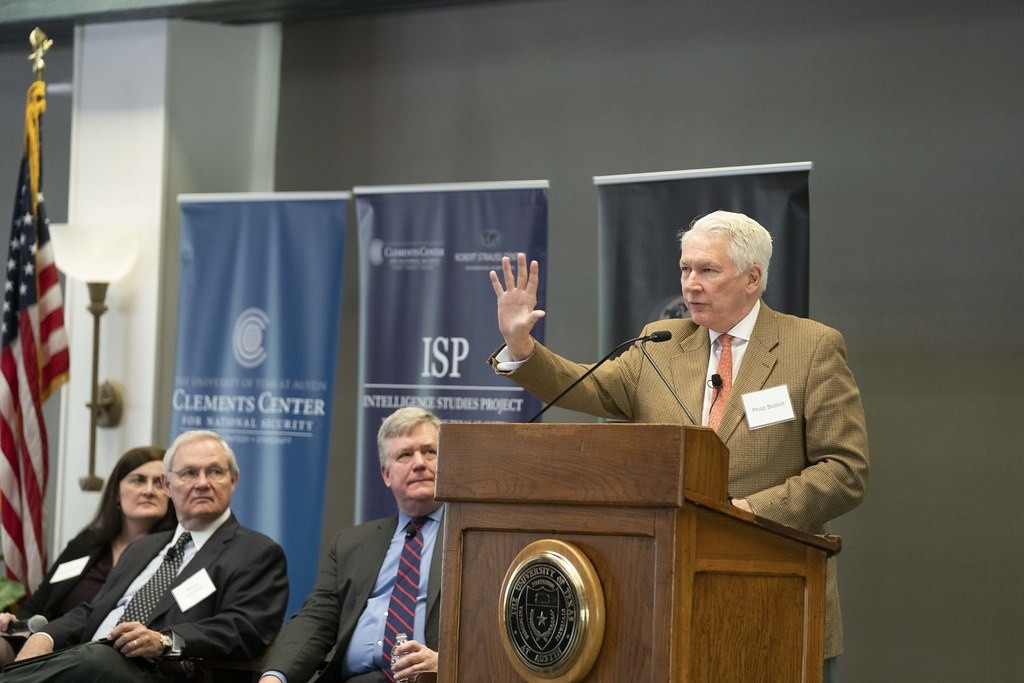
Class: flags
173,192,349,644
354,179,549,525
591,158,814,366
1,80,72,591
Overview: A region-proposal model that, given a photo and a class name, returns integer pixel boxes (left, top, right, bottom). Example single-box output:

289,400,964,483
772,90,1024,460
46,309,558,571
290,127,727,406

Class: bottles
390,633,420,683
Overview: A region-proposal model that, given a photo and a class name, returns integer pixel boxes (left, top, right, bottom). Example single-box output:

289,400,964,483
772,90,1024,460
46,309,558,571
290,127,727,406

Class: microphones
529,332,699,425
7,614,48,633
164,548,176,561
707,373,723,415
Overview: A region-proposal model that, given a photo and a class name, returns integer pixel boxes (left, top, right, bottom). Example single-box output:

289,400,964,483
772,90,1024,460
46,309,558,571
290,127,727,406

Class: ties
113,532,193,626
382,514,434,682
708,334,735,434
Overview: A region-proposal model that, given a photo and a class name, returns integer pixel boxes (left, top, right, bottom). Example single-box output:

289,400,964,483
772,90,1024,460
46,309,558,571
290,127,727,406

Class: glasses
169,466,231,482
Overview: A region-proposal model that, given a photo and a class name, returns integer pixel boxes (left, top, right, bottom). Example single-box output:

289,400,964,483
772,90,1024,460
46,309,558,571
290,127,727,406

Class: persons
0,427,292,683
485,206,872,683
1,447,178,667
256,406,446,683
